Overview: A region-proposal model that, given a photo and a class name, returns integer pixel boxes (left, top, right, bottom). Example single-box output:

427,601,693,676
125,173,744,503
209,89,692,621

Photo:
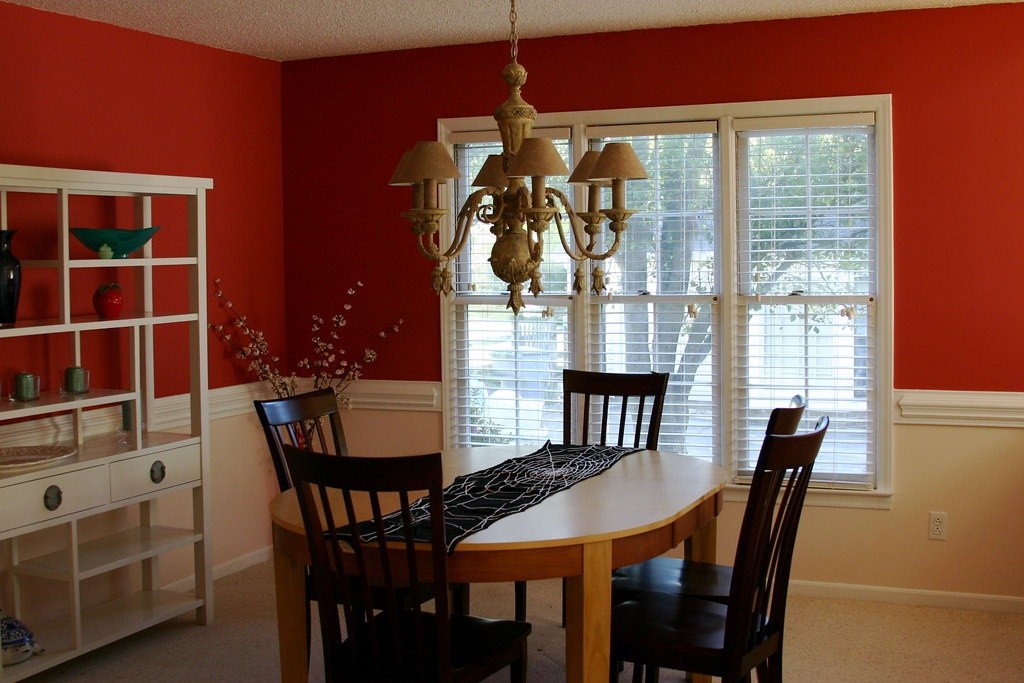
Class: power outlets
929,512,949,541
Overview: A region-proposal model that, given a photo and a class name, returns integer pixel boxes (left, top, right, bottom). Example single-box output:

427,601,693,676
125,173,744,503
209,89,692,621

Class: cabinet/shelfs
0,164,213,683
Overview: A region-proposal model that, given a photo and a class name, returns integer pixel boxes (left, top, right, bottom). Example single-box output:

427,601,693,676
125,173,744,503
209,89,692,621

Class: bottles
0,229,22,325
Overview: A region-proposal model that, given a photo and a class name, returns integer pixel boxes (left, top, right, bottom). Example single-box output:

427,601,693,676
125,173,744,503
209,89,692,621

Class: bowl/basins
69,225,161,258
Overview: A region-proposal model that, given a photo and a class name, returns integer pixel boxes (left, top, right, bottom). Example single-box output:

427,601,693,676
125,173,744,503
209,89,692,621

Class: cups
15,373,40,400
65,367,90,393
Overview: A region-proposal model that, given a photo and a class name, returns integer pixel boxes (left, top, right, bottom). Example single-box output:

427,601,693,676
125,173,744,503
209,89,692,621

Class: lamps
387,0,649,318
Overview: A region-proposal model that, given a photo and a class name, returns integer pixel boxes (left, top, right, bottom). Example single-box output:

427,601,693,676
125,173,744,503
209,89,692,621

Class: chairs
254,388,470,636
611,394,830,683
562,368,669,626
282,443,533,683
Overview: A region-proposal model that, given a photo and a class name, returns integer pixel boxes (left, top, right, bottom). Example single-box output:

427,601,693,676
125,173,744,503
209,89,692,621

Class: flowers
208,276,403,447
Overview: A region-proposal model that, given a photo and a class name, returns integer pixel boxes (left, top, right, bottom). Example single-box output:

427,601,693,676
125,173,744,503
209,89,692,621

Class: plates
0,445,78,469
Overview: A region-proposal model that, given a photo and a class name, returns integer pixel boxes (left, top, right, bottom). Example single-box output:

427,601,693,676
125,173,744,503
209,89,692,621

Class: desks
268,443,728,683
